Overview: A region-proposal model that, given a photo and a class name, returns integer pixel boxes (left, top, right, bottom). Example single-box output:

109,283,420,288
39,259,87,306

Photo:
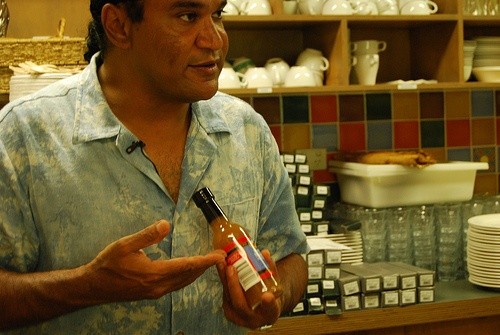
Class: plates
466,213,500,290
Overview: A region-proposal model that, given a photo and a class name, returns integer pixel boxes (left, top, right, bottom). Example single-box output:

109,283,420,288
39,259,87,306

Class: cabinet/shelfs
214,0,500,98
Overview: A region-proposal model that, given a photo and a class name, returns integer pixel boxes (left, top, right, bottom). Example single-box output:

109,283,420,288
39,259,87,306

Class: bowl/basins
463,35,500,83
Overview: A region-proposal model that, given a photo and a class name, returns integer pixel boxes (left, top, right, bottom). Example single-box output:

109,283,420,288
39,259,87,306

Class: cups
218,47,329,88
222,0,439,15
350,38,387,84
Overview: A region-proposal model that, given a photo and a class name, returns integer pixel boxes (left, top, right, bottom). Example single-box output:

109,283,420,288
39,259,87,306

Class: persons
0,0,310,335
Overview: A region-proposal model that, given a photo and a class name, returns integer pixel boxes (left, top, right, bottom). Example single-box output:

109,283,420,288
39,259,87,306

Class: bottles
191,187,279,310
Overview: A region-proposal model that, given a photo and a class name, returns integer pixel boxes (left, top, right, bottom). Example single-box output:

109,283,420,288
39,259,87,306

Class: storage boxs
332,158,489,210
